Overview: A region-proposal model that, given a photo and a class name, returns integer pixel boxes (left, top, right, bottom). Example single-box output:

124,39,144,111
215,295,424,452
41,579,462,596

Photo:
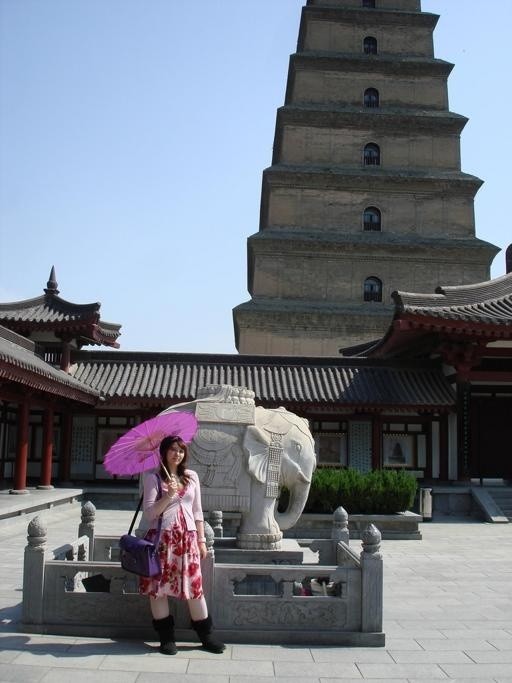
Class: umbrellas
102,411,198,480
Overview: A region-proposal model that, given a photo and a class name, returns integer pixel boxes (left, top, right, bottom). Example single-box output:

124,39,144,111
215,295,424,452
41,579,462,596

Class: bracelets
197,538,207,543
166,492,173,499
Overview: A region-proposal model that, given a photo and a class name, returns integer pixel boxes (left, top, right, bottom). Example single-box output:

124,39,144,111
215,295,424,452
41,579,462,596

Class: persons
135,436,226,654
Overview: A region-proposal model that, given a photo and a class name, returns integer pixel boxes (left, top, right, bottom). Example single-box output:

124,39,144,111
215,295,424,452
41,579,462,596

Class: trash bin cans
420,488,433,522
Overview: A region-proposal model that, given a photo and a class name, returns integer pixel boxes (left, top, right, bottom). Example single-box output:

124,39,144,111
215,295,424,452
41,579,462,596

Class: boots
191,615,225,653
152,615,178,654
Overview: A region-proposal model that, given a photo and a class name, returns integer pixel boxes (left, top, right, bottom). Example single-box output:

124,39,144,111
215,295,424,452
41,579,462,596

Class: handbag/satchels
119,535,160,576
